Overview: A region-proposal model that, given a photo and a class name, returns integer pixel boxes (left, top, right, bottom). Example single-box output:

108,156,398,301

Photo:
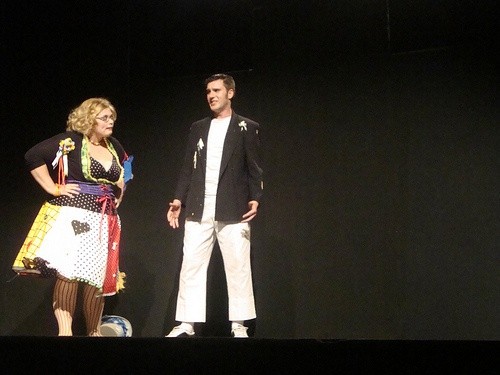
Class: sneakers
231,323,249,337
165,325,195,337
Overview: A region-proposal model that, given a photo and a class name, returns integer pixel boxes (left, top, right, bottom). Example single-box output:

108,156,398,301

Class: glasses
96,115,114,121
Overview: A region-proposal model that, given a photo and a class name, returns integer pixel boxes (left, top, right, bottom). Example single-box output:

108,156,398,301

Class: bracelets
55,183,61,197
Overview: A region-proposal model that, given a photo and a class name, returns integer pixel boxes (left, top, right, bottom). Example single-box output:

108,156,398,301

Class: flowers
239,120,248,132
51,138,75,185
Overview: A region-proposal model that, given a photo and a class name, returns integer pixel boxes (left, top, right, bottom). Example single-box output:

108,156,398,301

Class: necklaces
88,137,104,146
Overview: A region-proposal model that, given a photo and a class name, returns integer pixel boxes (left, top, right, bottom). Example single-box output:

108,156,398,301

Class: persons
12,97,130,336
166,74,267,338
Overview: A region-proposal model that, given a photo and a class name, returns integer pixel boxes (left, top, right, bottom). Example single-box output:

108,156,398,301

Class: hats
100,315,132,337
